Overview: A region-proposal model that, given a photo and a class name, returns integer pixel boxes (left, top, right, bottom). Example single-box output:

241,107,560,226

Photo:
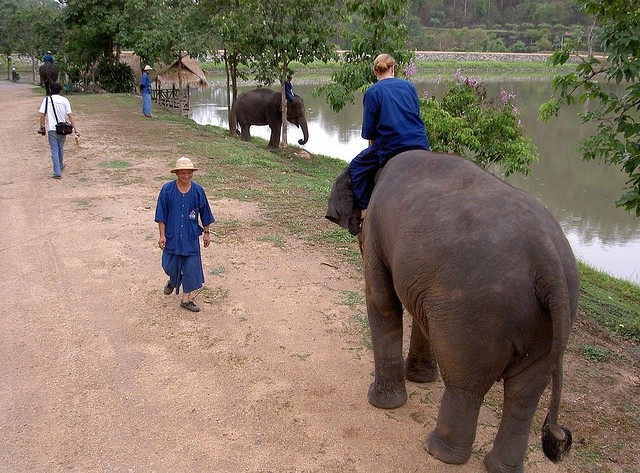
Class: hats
170,156,199,173
144,65,152,70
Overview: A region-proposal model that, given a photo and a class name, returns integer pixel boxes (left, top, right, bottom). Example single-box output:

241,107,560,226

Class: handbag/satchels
50,96,73,135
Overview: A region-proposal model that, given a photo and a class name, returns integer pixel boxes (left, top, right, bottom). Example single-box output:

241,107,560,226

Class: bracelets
204,230,209,233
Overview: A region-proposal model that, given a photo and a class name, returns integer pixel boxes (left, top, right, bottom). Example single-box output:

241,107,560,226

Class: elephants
231,86,310,149
323,145,584,473
38,60,60,96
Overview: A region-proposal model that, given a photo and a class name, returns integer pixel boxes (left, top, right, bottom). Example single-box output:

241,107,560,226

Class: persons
154,155,215,311
44,50,53,63
285,75,294,102
349,52,430,230
139,64,154,117
37,81,80,178
11,67,21,82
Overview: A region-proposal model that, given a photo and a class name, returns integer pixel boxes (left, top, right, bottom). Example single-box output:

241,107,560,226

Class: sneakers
164,283,174,295
180,299,200,312
